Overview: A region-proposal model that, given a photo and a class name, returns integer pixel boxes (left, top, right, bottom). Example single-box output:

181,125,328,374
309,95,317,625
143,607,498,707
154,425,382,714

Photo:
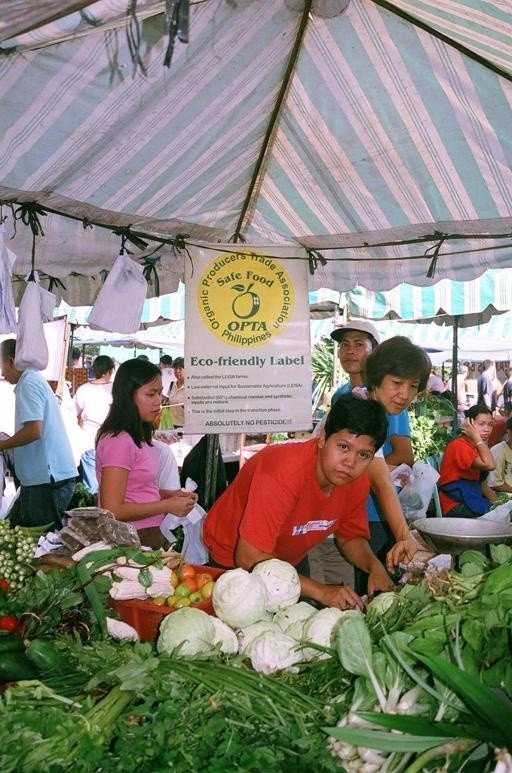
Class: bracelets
475,442,482,447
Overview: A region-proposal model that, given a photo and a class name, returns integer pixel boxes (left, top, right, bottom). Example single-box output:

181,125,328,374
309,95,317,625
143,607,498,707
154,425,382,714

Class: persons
427,366,444,393
307,335,431,604
329,320,414,597
73,356,114,449
66,346,88,396
447,359,511,424
482,419,512,500
95,359,198,551
438,404,497,517
159,355,184,429
218,433,244,483
202,393,394,613
1,339,80,528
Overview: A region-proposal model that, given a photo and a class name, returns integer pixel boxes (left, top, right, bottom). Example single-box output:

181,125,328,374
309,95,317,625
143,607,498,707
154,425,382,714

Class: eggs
145,558,408,673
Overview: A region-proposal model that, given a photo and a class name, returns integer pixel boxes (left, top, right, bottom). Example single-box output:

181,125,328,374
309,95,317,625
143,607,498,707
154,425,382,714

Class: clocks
0,667,139,773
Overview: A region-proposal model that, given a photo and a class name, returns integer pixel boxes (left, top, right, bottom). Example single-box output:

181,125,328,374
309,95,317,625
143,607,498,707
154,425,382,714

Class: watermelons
412,517,512,573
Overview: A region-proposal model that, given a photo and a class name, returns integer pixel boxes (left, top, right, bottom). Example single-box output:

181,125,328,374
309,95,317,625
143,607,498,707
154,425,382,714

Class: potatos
0,634,72,686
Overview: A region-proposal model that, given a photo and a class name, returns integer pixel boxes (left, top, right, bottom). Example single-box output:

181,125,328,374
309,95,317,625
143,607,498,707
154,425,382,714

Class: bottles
331,321,379,346
